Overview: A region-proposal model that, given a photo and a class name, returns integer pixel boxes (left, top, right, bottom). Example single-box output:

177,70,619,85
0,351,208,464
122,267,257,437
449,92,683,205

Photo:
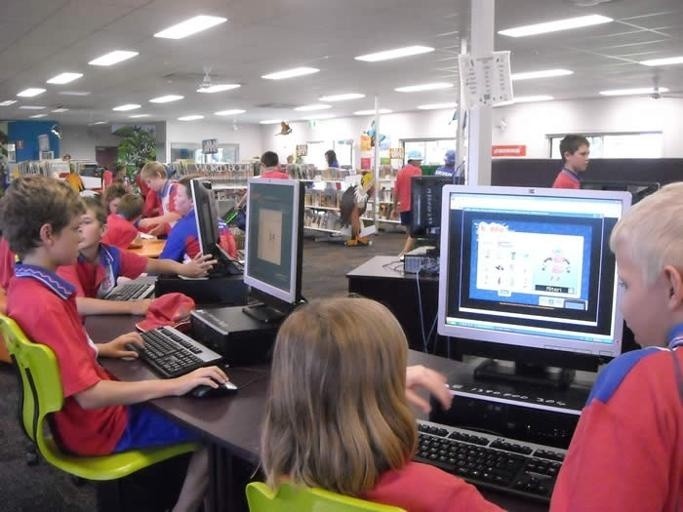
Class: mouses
192,381,238,398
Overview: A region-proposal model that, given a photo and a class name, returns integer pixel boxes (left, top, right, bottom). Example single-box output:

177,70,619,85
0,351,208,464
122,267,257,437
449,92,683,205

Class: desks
83,275,591,511
345,255,462,360
129,235,166,259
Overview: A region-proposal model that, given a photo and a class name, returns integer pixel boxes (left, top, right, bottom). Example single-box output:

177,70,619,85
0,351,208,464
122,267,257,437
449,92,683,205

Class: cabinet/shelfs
376,166,407,233
184,163,259,219
286,165,376,248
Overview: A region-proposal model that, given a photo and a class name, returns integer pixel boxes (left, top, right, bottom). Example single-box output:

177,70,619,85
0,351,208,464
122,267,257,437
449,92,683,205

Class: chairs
0,314,203,512
245,482,407,511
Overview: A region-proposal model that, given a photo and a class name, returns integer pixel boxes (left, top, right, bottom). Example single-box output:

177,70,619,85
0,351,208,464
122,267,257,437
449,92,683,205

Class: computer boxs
426,353,601,442
402,245,441,278
153,271,249,308
187,303,282,367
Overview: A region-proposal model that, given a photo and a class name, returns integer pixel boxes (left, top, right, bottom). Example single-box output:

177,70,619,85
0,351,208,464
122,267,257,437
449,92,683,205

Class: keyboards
410,418,568,506
125,326,223,379
104,283,156,301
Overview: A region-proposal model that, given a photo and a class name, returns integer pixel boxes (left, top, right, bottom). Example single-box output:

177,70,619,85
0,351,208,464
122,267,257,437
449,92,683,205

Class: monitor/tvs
189,180,220,262
436,184,633,388
410,176,464,256
242,176,304,323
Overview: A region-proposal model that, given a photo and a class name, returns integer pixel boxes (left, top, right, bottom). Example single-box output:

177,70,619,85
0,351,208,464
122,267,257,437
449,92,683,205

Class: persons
549,181,683,512
325,151,342,188
66,162,86,194
56,198,218,315
434,149,456,178
160,176,237,261
260,298,508,512
552,136,590,189
0,176,229,512
390,152,423,257
254,152,291,180
97,161,181,250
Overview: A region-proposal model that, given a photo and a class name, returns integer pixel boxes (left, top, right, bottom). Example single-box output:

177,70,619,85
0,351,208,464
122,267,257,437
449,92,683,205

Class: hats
134,292,196,335
406,150,425,162
